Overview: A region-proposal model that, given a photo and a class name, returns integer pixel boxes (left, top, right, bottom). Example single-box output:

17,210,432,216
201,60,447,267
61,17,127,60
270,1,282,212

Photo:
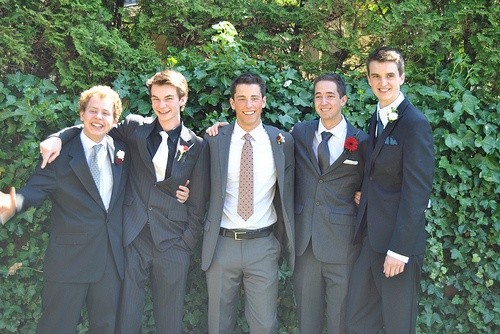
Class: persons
0,84,190,334
206,74,369,334
202,70,361,334
39,70,211,334
289,47,436,334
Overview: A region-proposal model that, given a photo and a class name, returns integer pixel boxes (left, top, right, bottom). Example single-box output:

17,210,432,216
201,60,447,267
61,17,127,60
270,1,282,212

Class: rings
40,151,43,154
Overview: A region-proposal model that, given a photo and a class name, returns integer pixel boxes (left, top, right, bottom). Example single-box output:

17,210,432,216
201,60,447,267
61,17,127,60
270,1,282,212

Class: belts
219,227,273,241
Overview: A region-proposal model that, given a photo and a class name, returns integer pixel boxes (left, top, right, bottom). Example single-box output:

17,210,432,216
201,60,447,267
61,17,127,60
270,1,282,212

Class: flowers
345,136,359,151
276,132,286,144
115,150,125,163
176,145,188,161
387,107,399,122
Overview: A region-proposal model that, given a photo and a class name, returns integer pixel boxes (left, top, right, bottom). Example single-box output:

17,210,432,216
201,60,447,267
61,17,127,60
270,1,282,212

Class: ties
90,144,103,190
377,111,384,138
152,131,169,182
237,132,254,222
317,131,333,175
